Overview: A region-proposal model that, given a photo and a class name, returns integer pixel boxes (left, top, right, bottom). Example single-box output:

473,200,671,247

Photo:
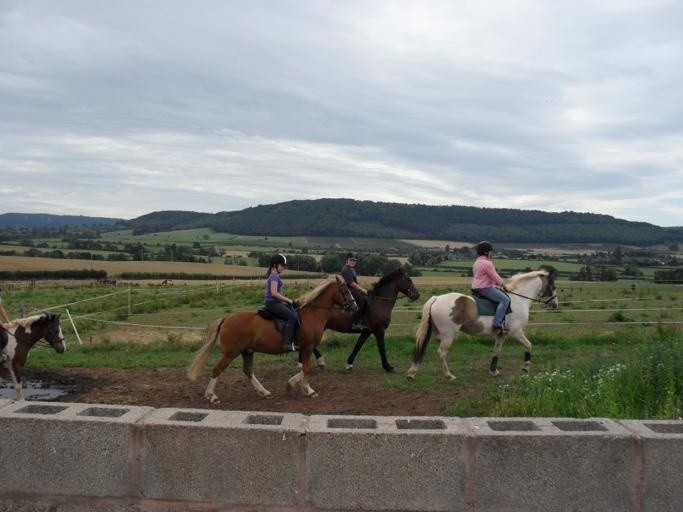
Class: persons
470,240,512,331
341,251,369,331
264,252,303,352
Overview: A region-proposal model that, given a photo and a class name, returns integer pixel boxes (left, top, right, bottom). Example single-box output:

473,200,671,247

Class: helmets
269,254,288,267
476,240,494,257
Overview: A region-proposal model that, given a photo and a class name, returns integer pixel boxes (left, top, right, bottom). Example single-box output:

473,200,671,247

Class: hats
346,252,359,261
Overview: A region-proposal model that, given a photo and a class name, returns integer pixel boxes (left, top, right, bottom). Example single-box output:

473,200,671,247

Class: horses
0,312,66,403
185,271,360,406
405,264,559,381
296,268,421,373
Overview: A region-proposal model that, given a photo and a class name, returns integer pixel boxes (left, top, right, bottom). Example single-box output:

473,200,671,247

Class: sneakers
282,342,300,352
490,325,510,335
350,323,369,331
